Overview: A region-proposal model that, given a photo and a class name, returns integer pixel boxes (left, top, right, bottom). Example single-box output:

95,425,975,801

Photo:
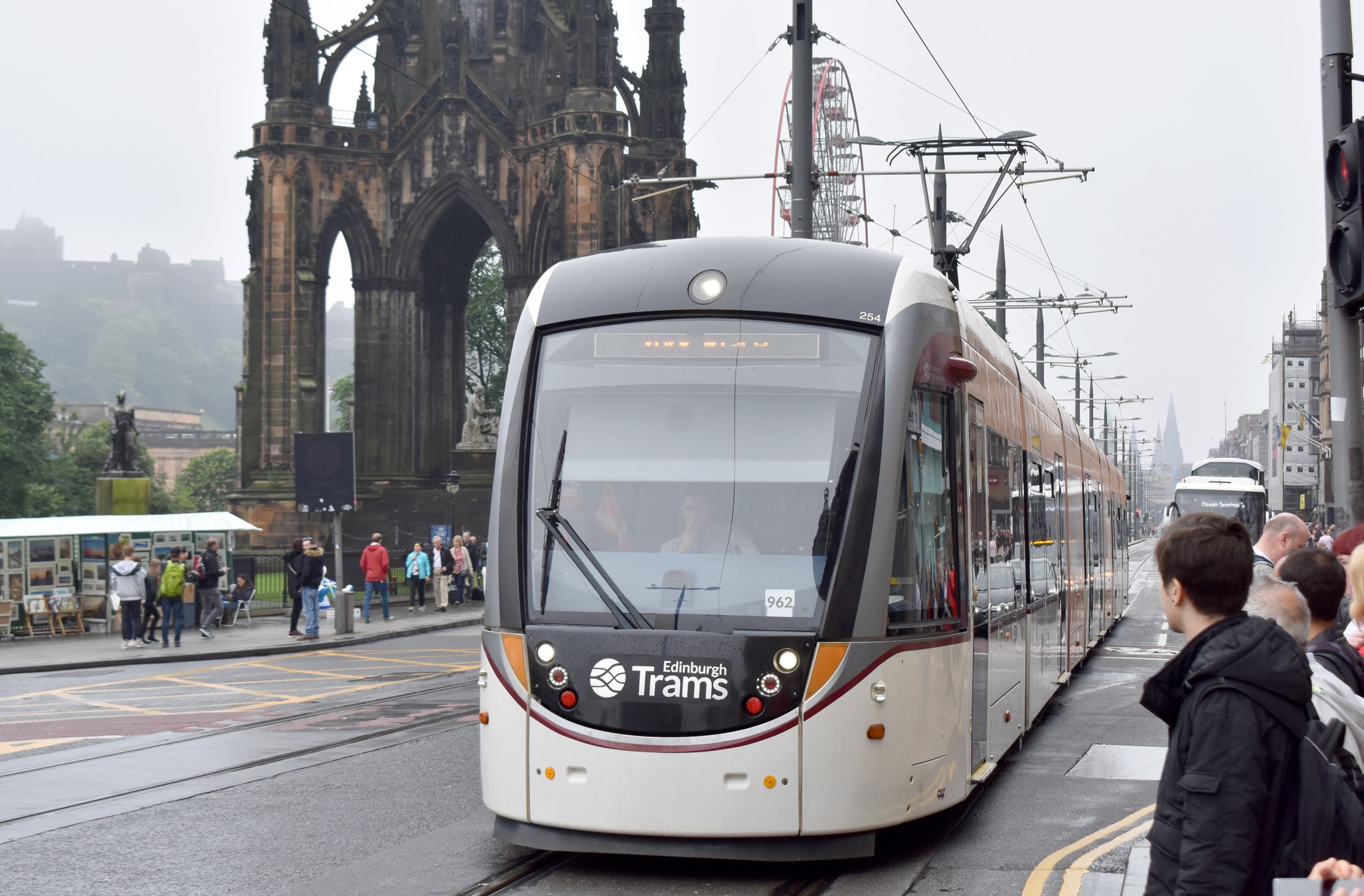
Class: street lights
445,470,461,549
1010,293,1158,544
845,129,1037,280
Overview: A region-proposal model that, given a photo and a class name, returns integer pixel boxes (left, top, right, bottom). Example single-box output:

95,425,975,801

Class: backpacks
189,554,207,584
161,561,185,598
1192,677,1364,880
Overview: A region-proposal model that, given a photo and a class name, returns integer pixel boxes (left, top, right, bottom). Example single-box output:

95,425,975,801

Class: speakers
293,432,356,512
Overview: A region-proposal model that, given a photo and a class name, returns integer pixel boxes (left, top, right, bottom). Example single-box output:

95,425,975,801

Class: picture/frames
0,532,229,622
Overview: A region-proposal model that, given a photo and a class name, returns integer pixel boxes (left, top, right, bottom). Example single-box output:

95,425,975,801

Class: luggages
448,581,458,605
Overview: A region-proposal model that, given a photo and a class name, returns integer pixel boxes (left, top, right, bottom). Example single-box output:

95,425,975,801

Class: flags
1276,423,1291,450
1289,400,1312,430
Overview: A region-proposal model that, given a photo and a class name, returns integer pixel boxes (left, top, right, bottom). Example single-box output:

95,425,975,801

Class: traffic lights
1135,509,1143,519
1323,119,1361,315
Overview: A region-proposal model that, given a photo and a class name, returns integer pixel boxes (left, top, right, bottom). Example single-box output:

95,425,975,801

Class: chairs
219,589,256,626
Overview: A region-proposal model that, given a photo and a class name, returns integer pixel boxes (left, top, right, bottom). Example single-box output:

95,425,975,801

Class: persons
1142,526,1145,536
450,532,488,607
427,536,456,611
661,488,759,555
283,538,304,636
111,546,189,649
1241,513,1364,774
405,542,430,612
972,529,1013,580
197,538,228,638
1148,526,1150,535
467,384,500,438
360,533,395,622
916,560,956,633
297,537,324,641
1307,857,1364,896
1139,513,1315,896
109,390,140,471
211,574,255,626
1152,526,1155,536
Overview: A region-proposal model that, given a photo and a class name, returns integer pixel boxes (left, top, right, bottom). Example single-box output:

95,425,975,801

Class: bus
470,123,1128,867
1166,458,1265,547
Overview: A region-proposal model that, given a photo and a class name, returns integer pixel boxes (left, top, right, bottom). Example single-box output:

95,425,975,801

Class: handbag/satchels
460,569,469,577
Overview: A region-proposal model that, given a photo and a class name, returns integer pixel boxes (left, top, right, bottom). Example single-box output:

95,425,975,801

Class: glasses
302,537,313,541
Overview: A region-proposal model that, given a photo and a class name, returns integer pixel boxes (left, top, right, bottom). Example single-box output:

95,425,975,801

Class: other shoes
419,605,425,610
409,606,414,611
162,643,168,647
441,606,446,611
313,634,319,639
201,633,210,638
199,628,216,638
120,636,160,649
459,602,464,606
364,618,371,622
288,629,305,637
175,642,181,646
298,634,314,640
435,607,441,611
456,600,460,605
383,615,395,621
